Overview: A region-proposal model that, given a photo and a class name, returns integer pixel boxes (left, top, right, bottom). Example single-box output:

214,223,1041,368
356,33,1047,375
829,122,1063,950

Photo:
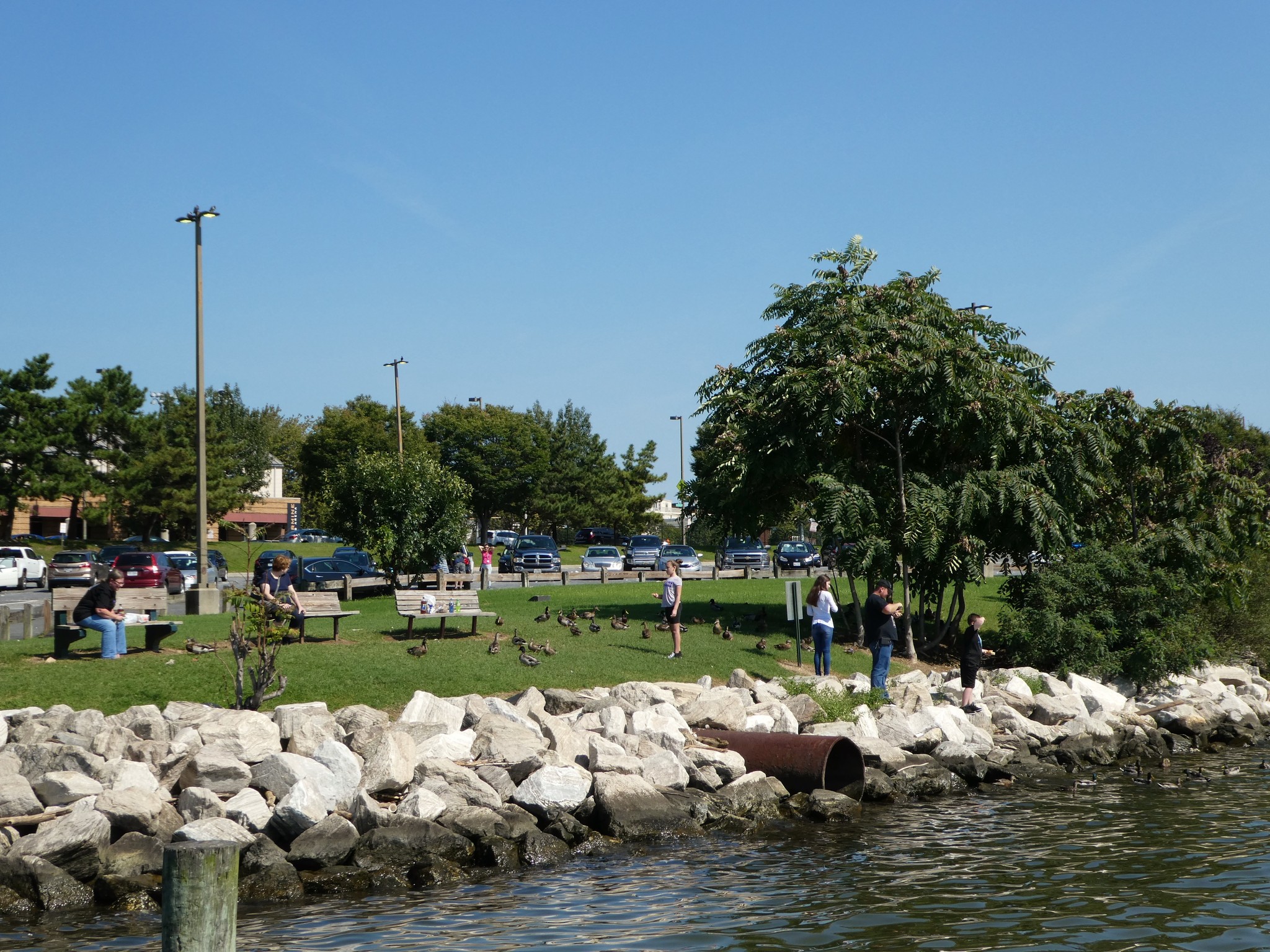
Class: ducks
639,621,651,639
756,638,767,650
713,606,768,640
578,606,600,620
511,628,558,656
495,616,504,627
557,608,577,628
182,637,218,662
801,635,860,655
588,616,601,633
516,646,541,667
691,616,705,624
405,639,429,658
773,639,793,651
488,632,502,655
708,598,723,612
609,609,632,631
566,620,583,637
533,607,550,623
993,759,1270,794
654,611,688,633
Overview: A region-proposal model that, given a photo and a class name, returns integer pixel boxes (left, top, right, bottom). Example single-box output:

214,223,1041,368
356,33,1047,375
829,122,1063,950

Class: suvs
417,543,474,590
820,537,856,571
505,535,566,574
715,535,772,579
574,527,631,546
476,529,536,547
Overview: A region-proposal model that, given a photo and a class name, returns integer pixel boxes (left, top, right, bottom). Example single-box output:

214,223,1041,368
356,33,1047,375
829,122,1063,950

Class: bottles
449,598,454,613
117,604,124,614
456,599,460,613
421,597,426,614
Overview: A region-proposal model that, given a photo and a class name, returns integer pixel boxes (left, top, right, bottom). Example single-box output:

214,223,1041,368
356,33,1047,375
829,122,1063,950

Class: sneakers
882,700,896,707
667,651,682,658
959,704,982,715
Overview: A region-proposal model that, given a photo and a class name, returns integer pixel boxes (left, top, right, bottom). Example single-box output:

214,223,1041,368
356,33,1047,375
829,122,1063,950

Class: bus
622,535,663,571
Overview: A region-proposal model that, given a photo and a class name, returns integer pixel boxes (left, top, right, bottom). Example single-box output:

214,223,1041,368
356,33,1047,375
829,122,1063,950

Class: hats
876,579,894,593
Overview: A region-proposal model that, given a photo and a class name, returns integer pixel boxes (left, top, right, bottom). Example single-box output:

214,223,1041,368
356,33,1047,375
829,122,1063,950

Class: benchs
261,592,359,645
51,588,183,659
393,590,497,642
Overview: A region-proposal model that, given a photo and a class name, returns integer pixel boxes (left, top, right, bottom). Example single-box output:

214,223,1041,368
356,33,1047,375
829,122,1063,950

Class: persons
434,553,449,591
652,558,683,659
806,574,838,676
257,555,306,642
72,570,127,660
959,613,993,715
862,580,903,707
451,552,467,591
478,544,495,590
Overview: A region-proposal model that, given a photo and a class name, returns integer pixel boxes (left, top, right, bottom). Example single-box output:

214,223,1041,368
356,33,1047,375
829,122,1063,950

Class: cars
497,548,512,574
45,535,81,541
252,549,299,585
804,542,821,568
0,544,228,596
579,546,626,572
279,528,347,543
772,541,815,570
10,534,45,541
122,536,168,542
268,557,391,599
654,544,703,580
331,547,380,573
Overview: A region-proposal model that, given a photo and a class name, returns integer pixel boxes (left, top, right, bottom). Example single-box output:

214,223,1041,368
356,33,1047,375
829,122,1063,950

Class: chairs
605,551,615,557
320,564,331,572
680,549,689,556
519,542,527,547
269,553,276,557
664,550,673,557
528,543,533,545
68,556,73,561
589,551,599,557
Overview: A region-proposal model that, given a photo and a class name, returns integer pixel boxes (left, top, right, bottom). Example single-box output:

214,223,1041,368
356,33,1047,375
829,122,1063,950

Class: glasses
113,580,124,584
287,566,290,569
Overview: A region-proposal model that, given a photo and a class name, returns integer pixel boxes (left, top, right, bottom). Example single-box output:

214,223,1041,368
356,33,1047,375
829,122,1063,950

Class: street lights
468,396,483,412
670,415,686,544
380,356,408,473
173,204,222,591
